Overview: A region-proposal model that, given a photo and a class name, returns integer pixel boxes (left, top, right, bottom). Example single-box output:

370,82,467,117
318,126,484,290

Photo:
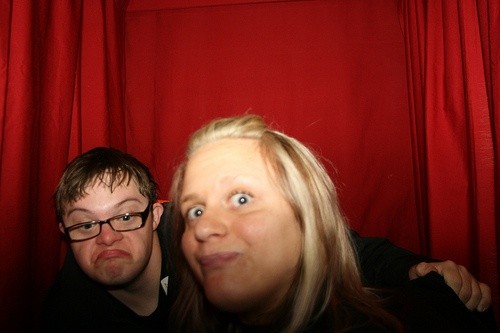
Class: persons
153,113,486,333
38,145,493,333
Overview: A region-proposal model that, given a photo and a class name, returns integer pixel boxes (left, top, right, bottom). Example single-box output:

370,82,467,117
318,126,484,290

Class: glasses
58,191,154,243
